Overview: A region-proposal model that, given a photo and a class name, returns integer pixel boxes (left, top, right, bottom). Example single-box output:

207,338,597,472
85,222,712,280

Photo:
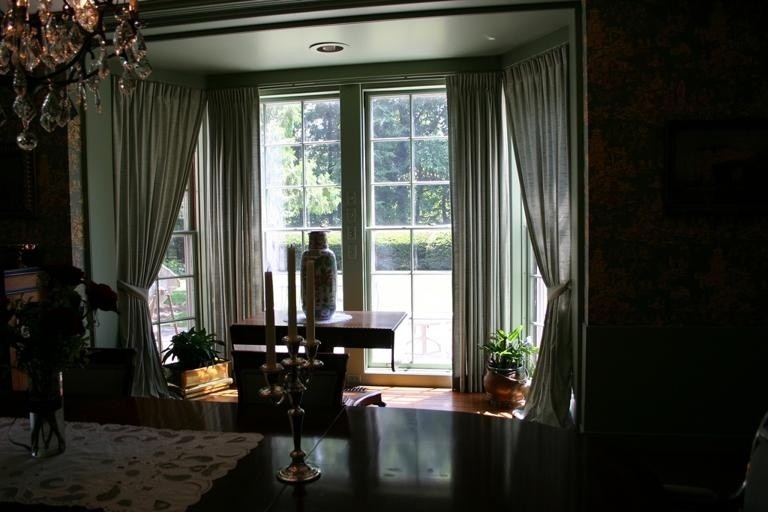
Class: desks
2,385,669,512
230,312,404,354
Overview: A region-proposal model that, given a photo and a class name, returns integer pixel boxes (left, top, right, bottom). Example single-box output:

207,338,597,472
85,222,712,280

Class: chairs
63,345,137,395
231,350,350,404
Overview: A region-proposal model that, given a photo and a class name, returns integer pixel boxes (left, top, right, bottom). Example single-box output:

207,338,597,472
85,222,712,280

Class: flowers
5,246,124,455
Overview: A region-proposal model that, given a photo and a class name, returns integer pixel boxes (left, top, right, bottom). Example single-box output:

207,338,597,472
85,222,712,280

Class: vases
300,232,337,321
26,372,66,458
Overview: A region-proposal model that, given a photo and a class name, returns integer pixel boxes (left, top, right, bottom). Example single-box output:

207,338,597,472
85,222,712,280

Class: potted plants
477,328,535,412
160,326,234,401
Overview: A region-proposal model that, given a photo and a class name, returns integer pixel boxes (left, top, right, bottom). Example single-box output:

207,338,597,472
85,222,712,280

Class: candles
264,243,316,368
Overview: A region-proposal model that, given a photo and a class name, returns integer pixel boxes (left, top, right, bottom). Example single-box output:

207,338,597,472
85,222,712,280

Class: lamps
0,0,155,152
